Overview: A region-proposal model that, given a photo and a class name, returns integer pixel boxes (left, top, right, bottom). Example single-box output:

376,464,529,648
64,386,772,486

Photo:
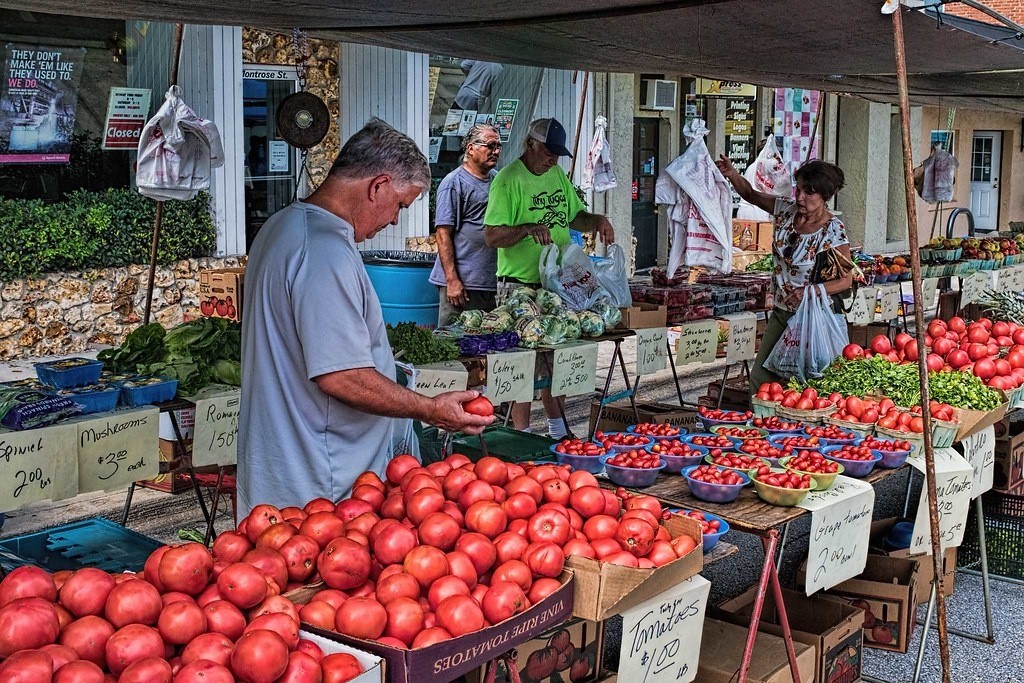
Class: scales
276,62,331,205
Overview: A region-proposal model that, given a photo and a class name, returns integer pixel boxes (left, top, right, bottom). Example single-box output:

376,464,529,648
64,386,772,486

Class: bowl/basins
750,418,808,435
514,460,575,477
644,441,709,475
697,409,753,433
704,451,771,488
679,432,744,455
666,509,730,553
709,424,769,443
597,422,687,487
748,467,818,506
817,445,883,478
592,431,656,455
766,432,828,452
874,272,909,283
778,455,845,492
681,465,751,503
853,437,915,469
550,443,615,475
735,441,799,465
800,424,861,445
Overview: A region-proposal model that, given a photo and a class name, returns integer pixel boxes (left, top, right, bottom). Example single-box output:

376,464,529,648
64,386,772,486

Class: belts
497,276,523,284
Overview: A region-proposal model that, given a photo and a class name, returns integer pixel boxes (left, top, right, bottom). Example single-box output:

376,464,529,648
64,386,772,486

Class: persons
429,123,502,328
714,154,853,412
236,116,496,528
483,117,615,443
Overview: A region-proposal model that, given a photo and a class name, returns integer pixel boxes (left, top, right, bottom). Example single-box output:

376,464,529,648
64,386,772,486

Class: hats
528,117,573,160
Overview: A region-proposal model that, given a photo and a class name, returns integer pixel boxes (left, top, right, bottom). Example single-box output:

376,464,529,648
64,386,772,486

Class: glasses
783,232,801,259
473,142,503,151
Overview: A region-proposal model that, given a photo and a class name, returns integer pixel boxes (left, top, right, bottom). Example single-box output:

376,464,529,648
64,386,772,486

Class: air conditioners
639,80,677,110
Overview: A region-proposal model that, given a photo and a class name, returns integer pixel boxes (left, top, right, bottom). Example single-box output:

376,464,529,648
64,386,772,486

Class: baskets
901,247,1024,280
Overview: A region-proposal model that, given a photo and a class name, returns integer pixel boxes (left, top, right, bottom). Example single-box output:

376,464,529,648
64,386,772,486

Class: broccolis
451,285,623,346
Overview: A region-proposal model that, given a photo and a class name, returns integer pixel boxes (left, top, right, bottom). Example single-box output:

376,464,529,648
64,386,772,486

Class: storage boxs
183,310,200,324
283,374,1024,683
620,303,667,329
875,300,915,316
33,357,198,495
451,426,556,465
941,291,997,322
200,268,247,322
0,515,168,582
668,218,790,355
912,244,1024,278
848,322,896,350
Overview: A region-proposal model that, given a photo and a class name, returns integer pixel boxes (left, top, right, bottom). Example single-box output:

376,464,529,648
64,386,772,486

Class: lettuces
386,320,461,365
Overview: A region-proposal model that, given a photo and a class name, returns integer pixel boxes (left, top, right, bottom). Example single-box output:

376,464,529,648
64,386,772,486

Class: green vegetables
745,253,774,271
788,354,1003,414
94,314,244,397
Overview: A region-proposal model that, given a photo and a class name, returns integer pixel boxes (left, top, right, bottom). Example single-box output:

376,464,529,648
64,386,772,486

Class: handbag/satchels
814,252,858,313
809,283,849,378
539,242,603,312
762,286,810,389
584,244,632,308
754,133,793,199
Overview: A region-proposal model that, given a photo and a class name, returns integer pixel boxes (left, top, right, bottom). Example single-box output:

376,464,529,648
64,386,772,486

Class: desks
594,469,999,683
461,308,625,440
609,309,773,425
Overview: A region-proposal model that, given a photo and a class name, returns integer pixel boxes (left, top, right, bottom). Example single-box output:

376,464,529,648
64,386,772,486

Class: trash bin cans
358,249,438,329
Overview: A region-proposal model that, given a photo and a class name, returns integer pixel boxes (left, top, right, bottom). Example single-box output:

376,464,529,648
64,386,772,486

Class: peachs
872,255,908,275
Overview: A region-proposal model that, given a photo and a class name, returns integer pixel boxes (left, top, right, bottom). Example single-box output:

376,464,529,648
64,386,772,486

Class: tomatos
215,453,699,653
842,317,1024,391
461,394,494,415
0,540,366,683
756,381,959,434
556,405,911,491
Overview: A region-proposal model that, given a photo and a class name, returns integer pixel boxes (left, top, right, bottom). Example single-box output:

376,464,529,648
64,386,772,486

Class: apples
929,235,1024,260
